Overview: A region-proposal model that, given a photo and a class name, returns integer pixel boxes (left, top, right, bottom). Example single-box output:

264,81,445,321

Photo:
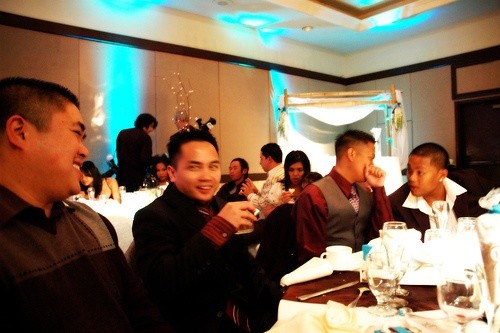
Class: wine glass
437,261,487,333
457,217,477,232
367,221,409,316
432,200,450,229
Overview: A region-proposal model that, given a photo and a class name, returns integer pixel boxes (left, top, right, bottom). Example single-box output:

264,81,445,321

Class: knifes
297,280,359,301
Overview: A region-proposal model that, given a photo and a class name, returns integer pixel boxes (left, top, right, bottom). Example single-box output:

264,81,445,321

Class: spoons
347,287,371,307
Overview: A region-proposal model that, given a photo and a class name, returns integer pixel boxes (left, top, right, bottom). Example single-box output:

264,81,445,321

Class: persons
153,156,171,185
132,127,258,333
268,150,311,206
388,143,488,243
116,113,158,192
79,161,121,204
240,143,285,219
291,130,395,265
0,76,137,333
216,157,249,201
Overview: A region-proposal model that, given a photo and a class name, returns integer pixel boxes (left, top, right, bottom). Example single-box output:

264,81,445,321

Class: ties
348,185,359,215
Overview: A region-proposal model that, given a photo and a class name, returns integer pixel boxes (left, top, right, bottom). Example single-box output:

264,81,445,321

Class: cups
320,245,352,268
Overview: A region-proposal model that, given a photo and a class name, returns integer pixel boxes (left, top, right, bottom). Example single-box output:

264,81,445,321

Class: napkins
281,256,331,287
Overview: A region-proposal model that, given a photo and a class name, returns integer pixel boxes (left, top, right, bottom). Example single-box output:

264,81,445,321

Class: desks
278,250,489,333
